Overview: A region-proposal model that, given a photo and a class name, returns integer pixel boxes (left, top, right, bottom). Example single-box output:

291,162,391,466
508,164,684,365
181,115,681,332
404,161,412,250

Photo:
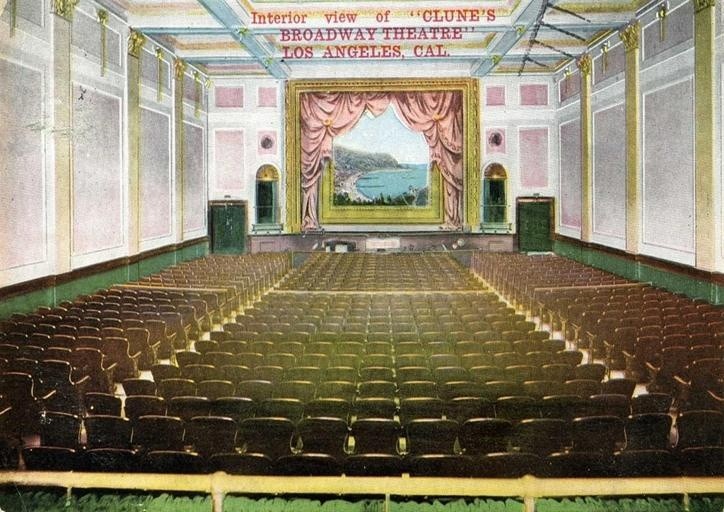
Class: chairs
1,249,724,478
